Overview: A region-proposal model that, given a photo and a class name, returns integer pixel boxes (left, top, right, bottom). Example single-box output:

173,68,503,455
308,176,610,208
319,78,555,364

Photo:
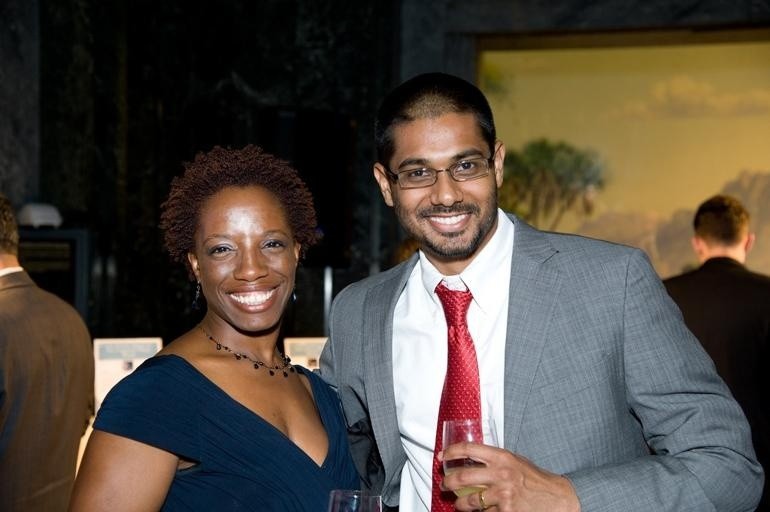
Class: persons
310,72,764,512
661,194,770,512
0,198,96,511
67,144,362,511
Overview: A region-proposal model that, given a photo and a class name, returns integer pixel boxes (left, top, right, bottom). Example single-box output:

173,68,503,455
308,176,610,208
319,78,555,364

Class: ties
432,282,483,512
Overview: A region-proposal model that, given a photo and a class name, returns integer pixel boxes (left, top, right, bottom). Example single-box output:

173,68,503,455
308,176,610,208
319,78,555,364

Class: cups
328,489,381,511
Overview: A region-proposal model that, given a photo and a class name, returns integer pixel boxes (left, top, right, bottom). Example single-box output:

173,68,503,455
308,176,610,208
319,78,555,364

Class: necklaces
196,324,294,378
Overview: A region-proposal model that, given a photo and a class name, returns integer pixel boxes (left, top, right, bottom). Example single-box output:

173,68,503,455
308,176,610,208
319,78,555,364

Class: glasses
385,150,494,189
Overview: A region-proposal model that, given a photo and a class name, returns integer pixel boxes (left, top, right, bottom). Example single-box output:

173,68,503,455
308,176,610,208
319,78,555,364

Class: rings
479,488,488,510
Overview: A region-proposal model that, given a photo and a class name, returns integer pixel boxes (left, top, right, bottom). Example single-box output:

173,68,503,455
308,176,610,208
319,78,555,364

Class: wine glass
442,418,498,511
